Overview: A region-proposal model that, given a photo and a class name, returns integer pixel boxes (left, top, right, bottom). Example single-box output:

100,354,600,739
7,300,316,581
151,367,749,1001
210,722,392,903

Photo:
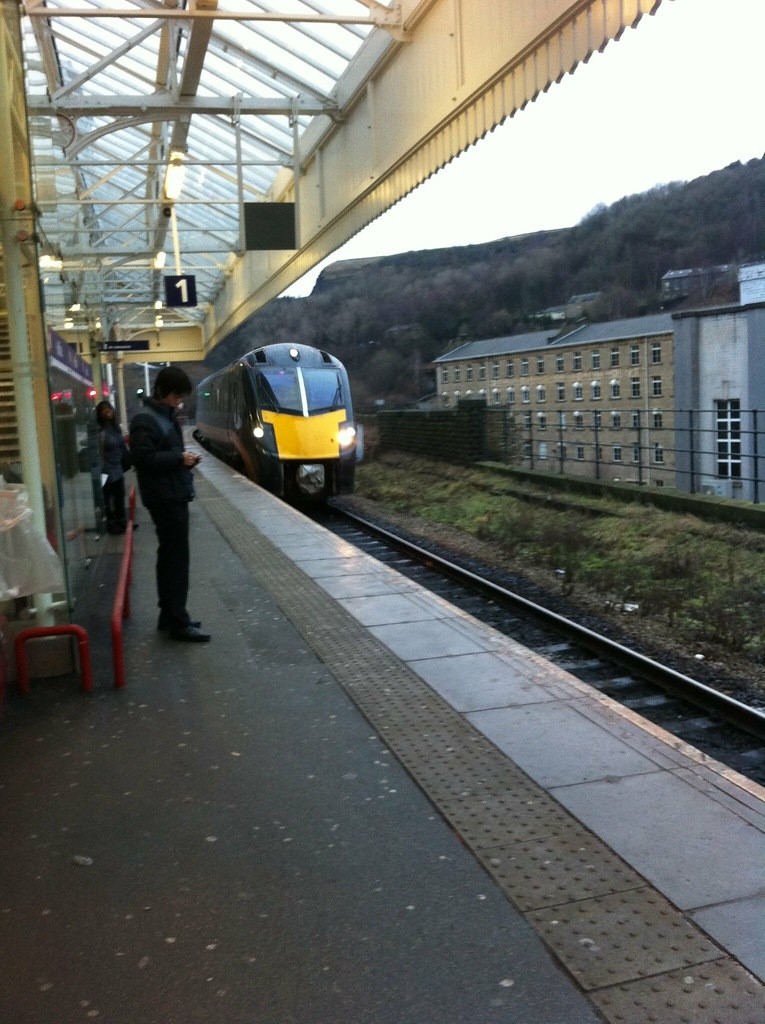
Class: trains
195,341,359,509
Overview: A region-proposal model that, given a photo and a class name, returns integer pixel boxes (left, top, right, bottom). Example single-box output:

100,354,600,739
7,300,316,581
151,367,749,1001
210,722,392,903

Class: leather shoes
187,618,201,626
159,625,210,641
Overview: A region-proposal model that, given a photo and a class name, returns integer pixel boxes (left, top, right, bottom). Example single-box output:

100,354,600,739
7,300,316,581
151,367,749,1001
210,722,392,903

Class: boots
121,517,138,529
107,520,125,534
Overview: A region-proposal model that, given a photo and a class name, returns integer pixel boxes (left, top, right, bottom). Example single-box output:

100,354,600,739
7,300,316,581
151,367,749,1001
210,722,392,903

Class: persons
96,400,139,534
128,366,210,643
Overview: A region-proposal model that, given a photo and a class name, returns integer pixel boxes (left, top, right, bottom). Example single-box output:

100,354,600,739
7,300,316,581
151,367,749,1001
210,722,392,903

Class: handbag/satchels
121,444,131,471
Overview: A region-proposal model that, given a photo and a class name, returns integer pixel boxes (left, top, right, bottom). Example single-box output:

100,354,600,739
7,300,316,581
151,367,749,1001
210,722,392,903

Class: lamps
162,151,190,200
